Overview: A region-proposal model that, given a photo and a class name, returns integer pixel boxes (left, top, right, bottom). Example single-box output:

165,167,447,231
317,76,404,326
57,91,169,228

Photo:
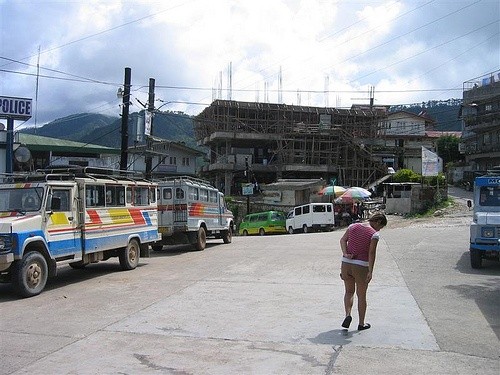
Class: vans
238,211,286,236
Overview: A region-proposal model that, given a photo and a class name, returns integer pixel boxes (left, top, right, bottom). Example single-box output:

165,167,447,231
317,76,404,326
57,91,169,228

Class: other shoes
358,323,371,330
342,316,352,328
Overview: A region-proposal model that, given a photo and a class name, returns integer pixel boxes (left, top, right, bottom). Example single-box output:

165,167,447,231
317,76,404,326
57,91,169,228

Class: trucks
285,203,335,234
466,173,500,266
157,176,236,249
0,167,163,295
